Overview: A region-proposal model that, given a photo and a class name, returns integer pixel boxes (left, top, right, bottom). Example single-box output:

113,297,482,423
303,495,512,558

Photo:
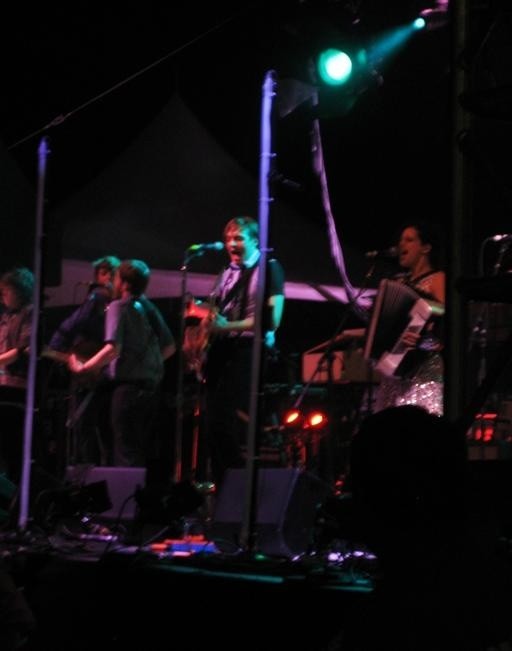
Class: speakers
83,466,147,521
212,467,325,559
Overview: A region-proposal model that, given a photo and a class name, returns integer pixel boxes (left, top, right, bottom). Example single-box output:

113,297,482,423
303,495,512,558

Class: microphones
82,281,113,291
190,241,225,251
366,247,400,257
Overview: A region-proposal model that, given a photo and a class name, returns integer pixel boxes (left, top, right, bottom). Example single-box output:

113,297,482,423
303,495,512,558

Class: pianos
302,329,379,384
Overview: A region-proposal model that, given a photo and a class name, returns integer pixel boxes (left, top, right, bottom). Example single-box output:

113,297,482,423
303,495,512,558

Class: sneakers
63,518,118,542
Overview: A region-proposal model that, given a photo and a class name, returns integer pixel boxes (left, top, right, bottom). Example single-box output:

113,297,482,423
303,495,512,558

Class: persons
49,255,122,470
71,257,177,468
359,223,447,421
183,213,287,481
0,267,36,487
